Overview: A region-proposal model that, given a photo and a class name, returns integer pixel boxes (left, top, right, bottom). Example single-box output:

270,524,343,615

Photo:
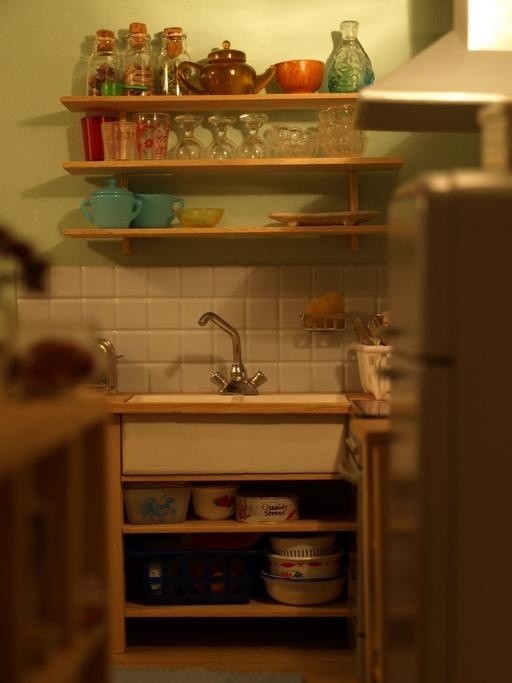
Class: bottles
88,18,191,98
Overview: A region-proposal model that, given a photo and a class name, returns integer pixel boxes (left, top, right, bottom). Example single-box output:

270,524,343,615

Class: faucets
198,310,268,393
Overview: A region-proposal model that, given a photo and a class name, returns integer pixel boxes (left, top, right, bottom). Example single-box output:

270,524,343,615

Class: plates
270,208,383,228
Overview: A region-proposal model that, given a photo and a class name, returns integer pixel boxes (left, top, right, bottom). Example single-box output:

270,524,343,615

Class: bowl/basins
274,57,325,95
190,481,241,521
274,103,366,157
259,572,349,605
266,552,345,579
175,205,225,226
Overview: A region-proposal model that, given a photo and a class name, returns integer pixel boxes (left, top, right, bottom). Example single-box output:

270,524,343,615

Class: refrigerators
382,170,509,682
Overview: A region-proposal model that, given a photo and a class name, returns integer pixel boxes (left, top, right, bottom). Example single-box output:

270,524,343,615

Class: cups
135,188,184,225
78,108,170,160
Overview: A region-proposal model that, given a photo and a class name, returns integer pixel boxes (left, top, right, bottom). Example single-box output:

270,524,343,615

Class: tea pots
178,37,274,96
78,175,138,229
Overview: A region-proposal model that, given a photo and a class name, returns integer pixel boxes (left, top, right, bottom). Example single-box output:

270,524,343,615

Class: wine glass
173,107,269,160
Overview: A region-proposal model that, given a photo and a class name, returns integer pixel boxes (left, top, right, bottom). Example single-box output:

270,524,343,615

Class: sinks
122,392,349,475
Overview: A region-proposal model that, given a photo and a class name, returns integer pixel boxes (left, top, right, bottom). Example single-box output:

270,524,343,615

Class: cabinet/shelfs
106,475,363,655
59,86,409,257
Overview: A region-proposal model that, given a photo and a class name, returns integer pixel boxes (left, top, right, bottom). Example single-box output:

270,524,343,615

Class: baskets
269,536,336,557
